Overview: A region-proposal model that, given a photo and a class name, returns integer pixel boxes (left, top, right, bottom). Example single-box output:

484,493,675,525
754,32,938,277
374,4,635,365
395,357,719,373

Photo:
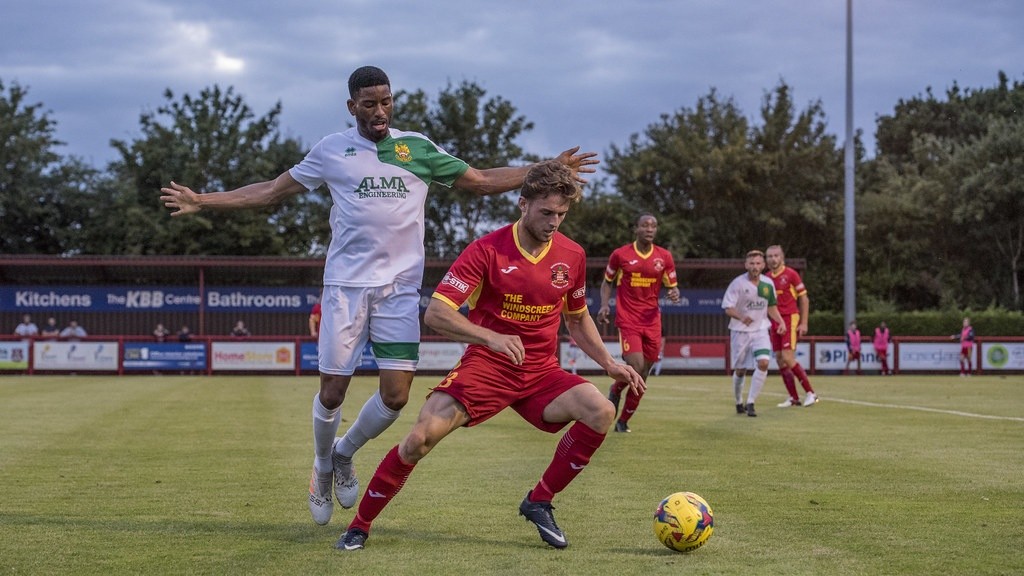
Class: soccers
653,492,714,553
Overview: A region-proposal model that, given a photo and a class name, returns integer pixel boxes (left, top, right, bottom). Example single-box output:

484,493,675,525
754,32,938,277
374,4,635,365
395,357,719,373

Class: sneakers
615,420,630,432
332,437,360,508
336,527,369,551
308,459,333,526
736,404,744,413
519,490,567,549
745,402,757,417
609,384,620,418
777,395,802,408
805,391,818,407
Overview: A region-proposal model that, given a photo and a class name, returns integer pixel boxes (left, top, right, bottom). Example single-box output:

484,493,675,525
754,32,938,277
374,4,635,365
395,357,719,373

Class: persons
844,320,864,375
655,327,665,375
60,320,86,337
873,321,893,375
597,212,680,433
951,318,974,376
43,317,60,335
231,320,250,336
178,324,192,335
14,313,38,335
764,245,820,407
309,288,323,336
160,66,600,526
335,160,647,551
722,250,786,417
152,324,170,337
